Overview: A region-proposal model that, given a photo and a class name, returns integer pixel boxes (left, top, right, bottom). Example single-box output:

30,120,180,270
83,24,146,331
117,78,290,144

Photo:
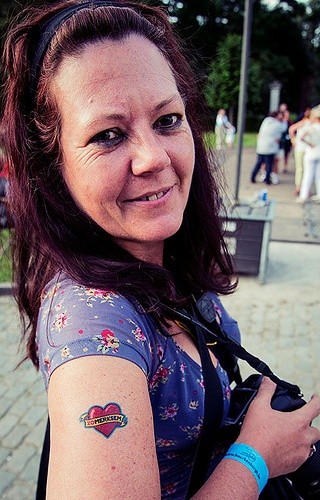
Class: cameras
222,371,312,450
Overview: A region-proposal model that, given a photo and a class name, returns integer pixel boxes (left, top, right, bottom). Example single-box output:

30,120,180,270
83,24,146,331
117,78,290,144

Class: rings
309,444,316,457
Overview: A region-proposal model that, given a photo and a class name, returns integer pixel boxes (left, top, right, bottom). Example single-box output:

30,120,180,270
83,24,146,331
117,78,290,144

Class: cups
262,192,267,201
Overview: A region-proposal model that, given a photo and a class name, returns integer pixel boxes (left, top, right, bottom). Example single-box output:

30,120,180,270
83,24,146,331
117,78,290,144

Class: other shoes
251,168,320,205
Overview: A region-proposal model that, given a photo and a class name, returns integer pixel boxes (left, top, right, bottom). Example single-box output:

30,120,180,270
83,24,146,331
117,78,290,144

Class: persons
226,128,235,149
214,109,233,150
280,110,293,174
257,113,286,184
297,105,320,204
3,0,318,500
278,104,288,114
289,108,312,195
251,112,287,185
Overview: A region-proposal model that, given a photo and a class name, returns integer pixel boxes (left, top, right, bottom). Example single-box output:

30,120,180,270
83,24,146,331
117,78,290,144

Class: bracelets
223,445,270,495
